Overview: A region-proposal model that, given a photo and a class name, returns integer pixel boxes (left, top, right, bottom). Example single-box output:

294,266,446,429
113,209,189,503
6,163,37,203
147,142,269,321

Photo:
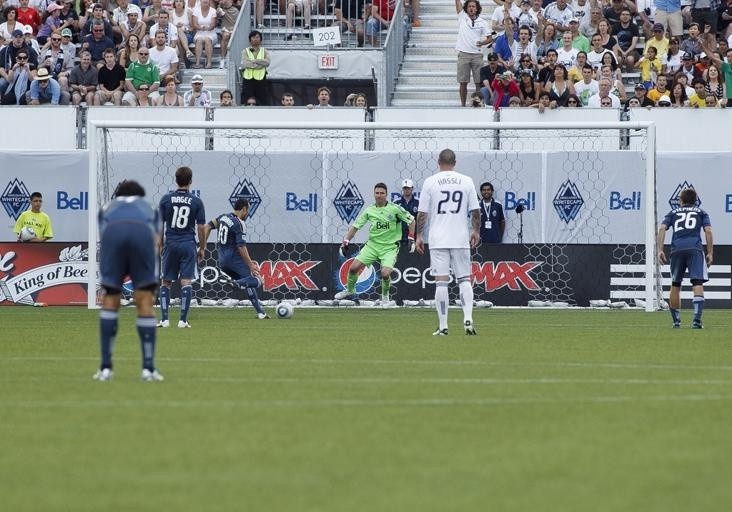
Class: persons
473,0,732,117
657,189,715,329
202,197,273,320
92,176,167,385
392,178,419,242
334,180,415,307
155,164,207,330
413,147,482,336
12,191,55,243
454,0,494,108
478,181,506,243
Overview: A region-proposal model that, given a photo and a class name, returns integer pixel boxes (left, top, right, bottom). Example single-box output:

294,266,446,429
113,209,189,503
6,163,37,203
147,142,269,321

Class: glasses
0,0,203,92
472,21,716,106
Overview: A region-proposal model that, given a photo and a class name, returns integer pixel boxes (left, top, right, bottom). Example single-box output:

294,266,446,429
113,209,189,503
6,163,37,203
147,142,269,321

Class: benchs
396,0,732,107
249,15,338,37
1,0,239,104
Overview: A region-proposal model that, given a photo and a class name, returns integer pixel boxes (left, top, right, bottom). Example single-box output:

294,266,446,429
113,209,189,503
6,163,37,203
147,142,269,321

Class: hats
400,179,413,189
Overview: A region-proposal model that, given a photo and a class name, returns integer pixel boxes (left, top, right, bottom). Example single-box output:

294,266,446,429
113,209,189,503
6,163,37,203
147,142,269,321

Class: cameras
497,74,507,80
18,63,25,66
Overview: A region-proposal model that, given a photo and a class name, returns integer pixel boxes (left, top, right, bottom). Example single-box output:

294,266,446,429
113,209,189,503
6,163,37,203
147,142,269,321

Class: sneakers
463,320,478,336
255,312,271,320
177,320,192,329
334,288,356,301
155,320,170,328
228,280,245,293
91,367,113,383
381,293,390,310
140,368,164,383
431,327,449,338
671,321,681,329
691,319,705,329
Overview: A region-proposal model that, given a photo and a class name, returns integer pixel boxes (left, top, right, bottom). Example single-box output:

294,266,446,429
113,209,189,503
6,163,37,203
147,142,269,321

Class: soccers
276,302,293,319
19,227,34,242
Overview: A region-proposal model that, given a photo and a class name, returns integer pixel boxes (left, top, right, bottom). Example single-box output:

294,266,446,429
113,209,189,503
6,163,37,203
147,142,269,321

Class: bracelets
341,239,350,245
407,233,415,241
